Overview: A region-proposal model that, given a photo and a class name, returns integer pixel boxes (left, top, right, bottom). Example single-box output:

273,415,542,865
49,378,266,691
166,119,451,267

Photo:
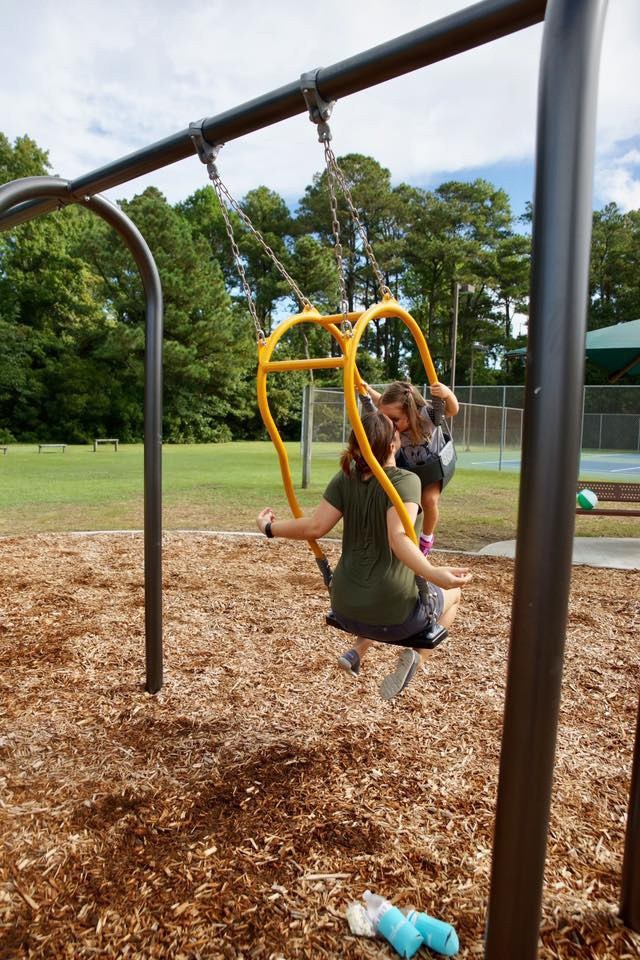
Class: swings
207,123,450,649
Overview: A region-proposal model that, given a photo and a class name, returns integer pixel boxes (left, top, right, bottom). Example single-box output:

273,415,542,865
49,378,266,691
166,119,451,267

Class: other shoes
419,535,434,557
337,650,360,679
380,649,419,700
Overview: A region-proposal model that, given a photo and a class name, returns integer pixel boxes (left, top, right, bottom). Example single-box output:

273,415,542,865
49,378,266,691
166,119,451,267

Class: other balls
578,487,598,509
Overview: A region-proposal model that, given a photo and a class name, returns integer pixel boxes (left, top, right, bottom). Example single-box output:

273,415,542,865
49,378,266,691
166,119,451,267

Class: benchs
574,480,640,517
39,444,67,454
92,439,119,452
0,447,7,455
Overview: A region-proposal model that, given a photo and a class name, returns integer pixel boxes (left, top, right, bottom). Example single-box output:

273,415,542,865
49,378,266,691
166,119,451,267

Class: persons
354,380,459,556
255,413,472,701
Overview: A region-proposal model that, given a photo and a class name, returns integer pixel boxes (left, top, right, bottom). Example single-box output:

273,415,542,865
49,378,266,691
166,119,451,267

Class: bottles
399,909,459,956
362,890,423,959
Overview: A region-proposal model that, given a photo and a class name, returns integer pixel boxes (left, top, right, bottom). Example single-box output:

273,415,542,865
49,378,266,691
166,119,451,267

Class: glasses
390,421,399,442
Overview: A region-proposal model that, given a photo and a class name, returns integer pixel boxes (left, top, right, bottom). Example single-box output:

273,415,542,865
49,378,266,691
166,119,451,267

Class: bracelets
265,524,274,538
368,388,371,392
